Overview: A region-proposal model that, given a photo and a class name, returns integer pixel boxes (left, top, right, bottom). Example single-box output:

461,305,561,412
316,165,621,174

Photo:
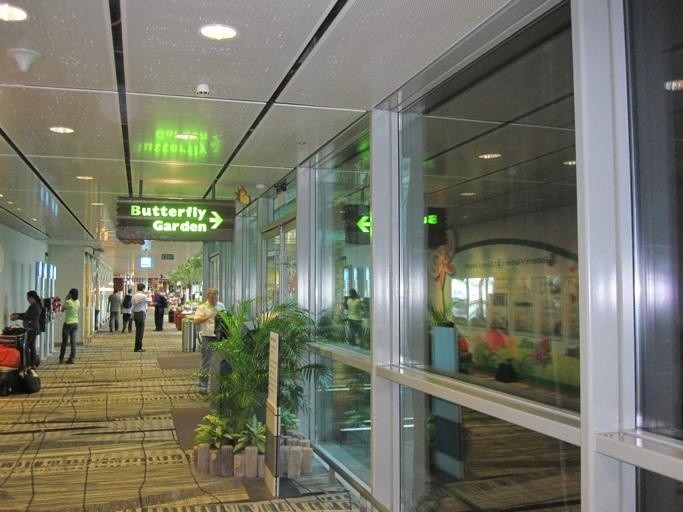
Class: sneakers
65,359,74,364
59,359,63,363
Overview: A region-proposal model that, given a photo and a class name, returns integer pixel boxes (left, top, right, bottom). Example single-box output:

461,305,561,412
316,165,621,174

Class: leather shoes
134,348,145,352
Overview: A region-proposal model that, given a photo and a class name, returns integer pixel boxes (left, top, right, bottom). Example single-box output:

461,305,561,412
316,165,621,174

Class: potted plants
157,258,202,328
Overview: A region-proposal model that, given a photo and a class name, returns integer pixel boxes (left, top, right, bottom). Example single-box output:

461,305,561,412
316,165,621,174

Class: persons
131,283,152,352
121,289,134,333
59,289,80,363
345,289,364,346
150,290,164,331
107,289,122,332
193,288,225,395
10,291,42,370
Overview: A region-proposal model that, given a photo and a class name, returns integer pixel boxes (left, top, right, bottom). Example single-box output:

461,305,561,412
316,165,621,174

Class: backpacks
123,295,133,308
159,295,168,307
0,367,41,396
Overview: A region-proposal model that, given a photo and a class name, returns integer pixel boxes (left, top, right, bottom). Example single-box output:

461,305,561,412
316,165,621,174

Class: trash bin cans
182,320,196,352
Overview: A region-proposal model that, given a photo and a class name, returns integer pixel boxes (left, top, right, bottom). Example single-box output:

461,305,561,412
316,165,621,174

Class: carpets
157,354,203,370
171,405,212,449
